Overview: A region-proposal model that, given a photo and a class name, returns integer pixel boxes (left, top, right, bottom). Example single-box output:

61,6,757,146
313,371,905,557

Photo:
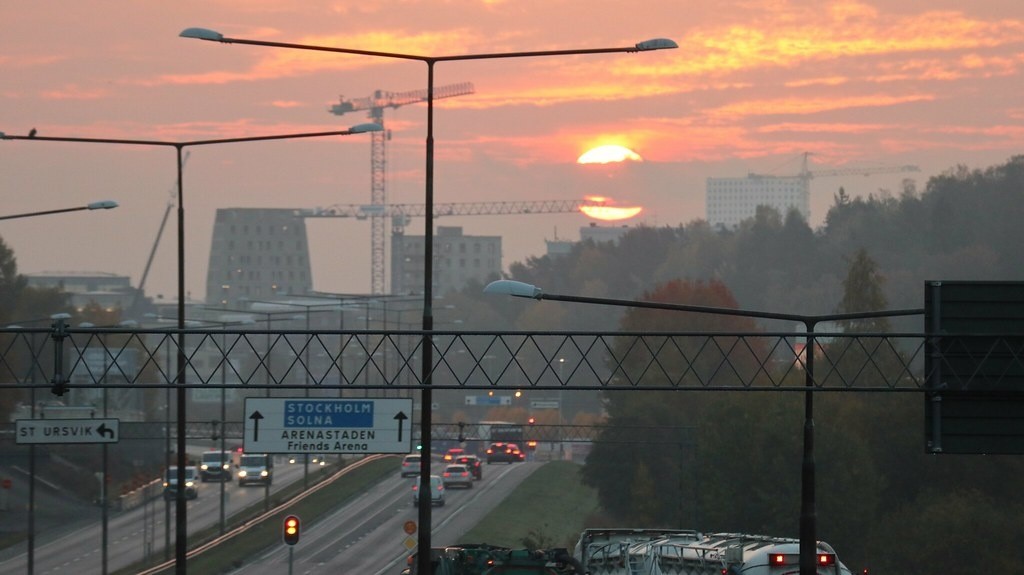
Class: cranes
289,197,592,294
329,81,477,299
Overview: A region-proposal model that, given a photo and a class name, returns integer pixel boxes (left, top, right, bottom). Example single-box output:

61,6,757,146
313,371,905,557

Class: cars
441,463,474,488
412,475,447,507
400,454,423,479
452,454,483,480
443,448,465,466
485,442,525,464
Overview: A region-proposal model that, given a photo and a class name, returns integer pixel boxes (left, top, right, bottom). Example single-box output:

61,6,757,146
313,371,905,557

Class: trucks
400,542,584,575
572,527,856,575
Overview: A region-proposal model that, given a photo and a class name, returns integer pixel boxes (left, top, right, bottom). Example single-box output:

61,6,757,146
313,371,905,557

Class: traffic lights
283,515,300,546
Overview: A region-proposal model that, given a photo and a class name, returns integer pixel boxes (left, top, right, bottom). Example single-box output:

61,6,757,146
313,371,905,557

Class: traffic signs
242,395,414,454
15,418,120,444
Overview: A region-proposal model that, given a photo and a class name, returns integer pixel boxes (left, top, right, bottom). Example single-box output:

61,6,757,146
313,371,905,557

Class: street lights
178,27,679,575
0,123,391,575
484,280,925,575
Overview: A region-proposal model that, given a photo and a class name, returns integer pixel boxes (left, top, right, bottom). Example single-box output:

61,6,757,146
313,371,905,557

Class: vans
236,453,274,487
198,449,234,481
163,464,200,500
